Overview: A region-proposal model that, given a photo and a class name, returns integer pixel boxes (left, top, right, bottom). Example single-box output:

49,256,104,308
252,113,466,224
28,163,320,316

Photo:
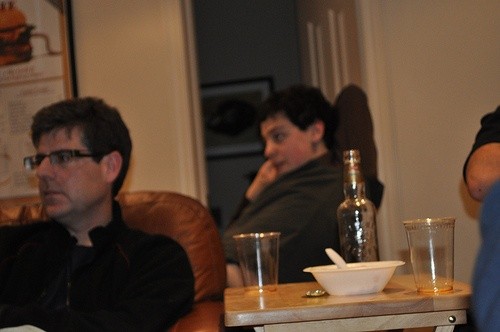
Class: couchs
0,190,222,332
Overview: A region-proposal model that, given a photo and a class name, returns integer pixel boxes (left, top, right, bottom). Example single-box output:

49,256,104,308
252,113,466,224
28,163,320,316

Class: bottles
336,149,380,264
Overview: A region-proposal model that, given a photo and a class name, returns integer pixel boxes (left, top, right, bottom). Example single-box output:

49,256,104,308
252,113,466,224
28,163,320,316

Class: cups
403,216,456,294
233,232,281,296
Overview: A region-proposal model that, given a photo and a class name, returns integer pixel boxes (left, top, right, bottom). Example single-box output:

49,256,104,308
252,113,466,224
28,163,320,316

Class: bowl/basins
303,261,406,296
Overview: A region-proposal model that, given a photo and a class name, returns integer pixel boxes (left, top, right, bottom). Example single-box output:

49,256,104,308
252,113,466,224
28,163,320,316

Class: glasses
23,150,95,172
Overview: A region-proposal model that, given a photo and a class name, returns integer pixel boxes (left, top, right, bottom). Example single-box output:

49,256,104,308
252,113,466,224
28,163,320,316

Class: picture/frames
200,75,274,161
0,0,73,197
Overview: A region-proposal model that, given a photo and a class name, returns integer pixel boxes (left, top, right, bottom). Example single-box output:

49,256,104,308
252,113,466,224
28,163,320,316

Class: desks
225,276,472,332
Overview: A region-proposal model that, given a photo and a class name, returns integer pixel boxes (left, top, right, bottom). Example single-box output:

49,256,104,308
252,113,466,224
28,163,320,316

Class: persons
218,85,385,284
462,106,500,201
0,97,196,332
